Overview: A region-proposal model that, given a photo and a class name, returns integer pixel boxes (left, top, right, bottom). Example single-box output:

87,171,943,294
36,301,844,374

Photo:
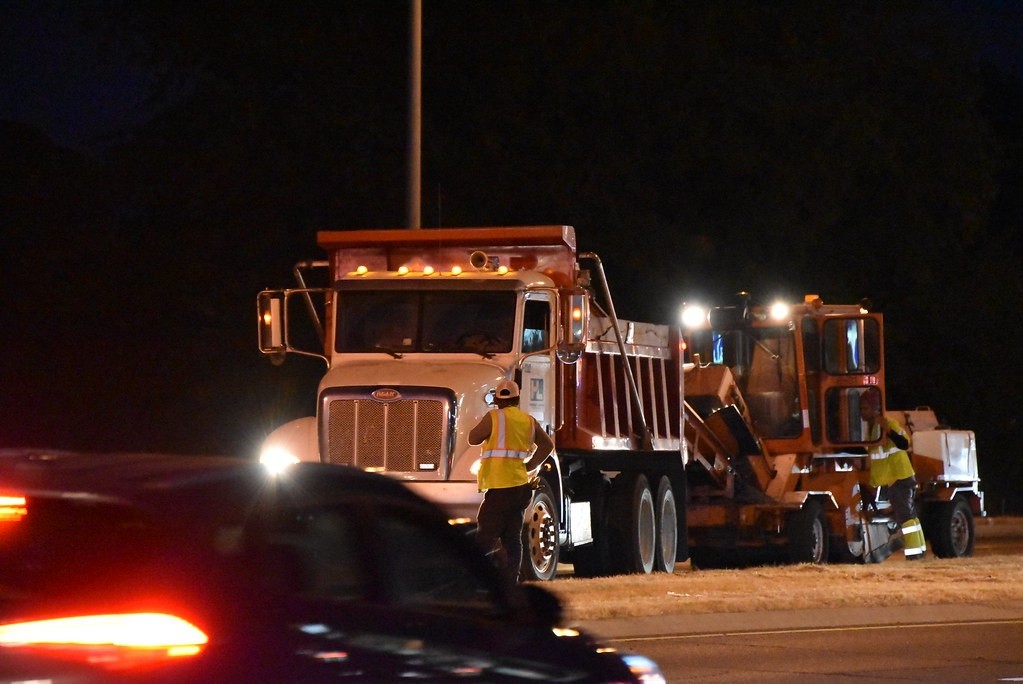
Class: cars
0,450,671,684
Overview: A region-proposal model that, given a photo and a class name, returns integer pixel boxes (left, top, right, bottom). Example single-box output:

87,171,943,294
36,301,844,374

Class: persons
858,389,927,561
467,380,555,584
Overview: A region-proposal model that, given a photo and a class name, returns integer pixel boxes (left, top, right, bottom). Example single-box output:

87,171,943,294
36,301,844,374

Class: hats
496,380,518,399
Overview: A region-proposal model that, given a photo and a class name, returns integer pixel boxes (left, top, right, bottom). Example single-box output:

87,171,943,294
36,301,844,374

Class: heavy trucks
250,222,693,571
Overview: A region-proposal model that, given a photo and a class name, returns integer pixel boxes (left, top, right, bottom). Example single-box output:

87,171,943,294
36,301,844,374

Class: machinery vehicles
682,290,992,560
